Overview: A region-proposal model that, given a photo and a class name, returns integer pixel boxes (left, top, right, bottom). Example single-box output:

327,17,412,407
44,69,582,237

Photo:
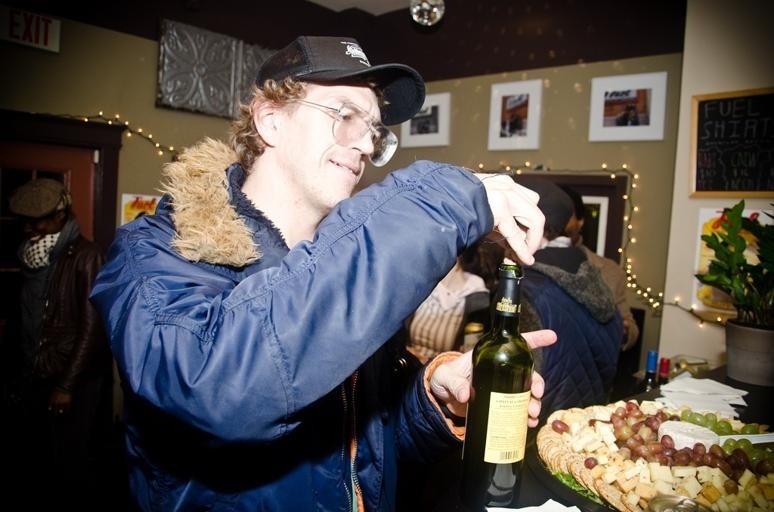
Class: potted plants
695,199,774,386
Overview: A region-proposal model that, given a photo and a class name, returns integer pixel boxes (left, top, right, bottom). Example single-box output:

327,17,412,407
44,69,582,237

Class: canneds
464,323,483,351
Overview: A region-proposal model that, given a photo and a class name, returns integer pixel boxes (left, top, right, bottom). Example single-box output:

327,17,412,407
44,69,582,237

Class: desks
487,364,774,512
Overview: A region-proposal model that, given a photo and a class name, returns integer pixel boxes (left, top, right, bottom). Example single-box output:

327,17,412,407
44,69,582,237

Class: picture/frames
588,71,667,142
487,79,543,151
400,93,451,149
497,173,628,270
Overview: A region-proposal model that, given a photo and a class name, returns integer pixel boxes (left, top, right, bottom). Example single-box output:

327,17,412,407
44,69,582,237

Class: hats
514,174,574,234
251,35,426,126
9,178,72,219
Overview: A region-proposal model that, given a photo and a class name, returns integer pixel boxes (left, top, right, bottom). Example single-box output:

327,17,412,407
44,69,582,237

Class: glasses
281,92,400,168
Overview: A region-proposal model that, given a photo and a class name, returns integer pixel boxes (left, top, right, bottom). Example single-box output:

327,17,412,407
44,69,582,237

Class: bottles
457,261,536,510
639,350,670,392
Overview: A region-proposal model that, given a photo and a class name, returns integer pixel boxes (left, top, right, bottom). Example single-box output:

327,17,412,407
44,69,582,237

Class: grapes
552,403,774,494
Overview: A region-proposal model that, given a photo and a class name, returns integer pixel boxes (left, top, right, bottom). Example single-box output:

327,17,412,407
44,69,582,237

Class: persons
561,185,640,350
90,35,559,511
0,177,114,511
486,174,624,446
405,253,485,367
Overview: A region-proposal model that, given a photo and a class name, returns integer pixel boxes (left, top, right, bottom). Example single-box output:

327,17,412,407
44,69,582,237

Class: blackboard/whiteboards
688,86,774,200
